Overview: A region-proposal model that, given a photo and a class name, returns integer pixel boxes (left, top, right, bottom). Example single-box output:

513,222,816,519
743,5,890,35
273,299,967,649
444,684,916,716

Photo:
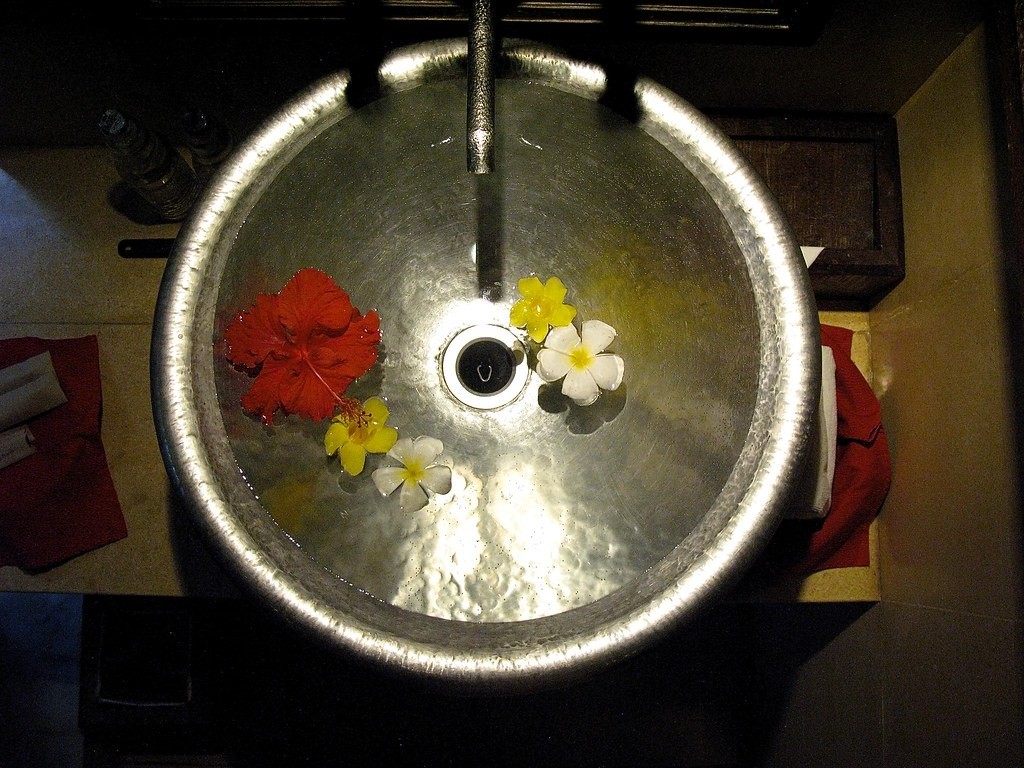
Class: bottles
98,110,200,219
178,109,233,166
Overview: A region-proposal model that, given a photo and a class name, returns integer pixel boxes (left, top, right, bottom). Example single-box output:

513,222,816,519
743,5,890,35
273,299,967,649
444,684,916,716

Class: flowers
371,435,452,514
538,320,624,407
509,276,578,344
324,396,397,477
224,267,383,428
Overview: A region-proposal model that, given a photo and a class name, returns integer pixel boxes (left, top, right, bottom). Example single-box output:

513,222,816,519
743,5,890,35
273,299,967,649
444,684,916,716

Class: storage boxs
711,114,905,311
